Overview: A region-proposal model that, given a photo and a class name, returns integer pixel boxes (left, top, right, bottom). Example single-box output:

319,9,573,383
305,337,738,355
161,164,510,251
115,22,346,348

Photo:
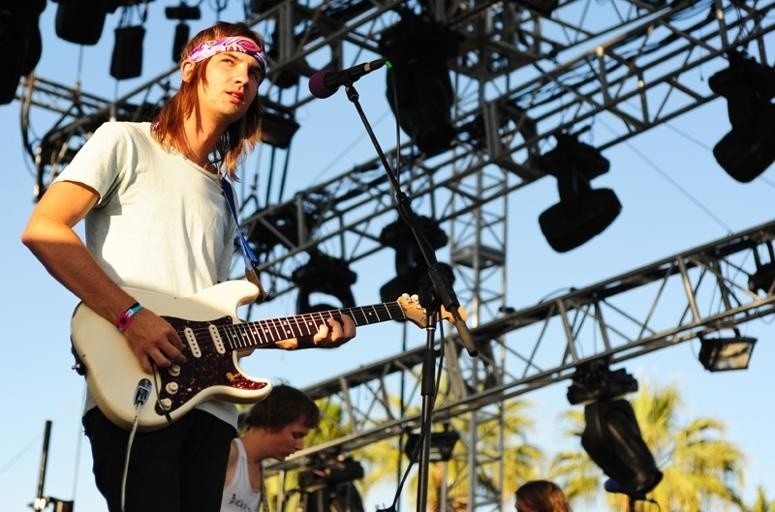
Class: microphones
306,57,390,100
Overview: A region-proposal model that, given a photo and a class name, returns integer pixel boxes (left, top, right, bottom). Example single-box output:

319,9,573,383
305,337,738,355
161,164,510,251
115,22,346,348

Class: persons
21,20,356,512
221,382,320,512
513,480,571,512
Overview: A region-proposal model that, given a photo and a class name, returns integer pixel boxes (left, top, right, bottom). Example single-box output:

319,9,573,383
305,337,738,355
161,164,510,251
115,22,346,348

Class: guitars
69,280,466,434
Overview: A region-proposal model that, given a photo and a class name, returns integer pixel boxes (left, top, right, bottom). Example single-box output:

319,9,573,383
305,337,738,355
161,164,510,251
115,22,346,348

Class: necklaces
202,161,211,170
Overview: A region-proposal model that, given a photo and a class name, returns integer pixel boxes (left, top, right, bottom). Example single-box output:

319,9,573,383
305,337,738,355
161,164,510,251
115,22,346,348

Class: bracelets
114,305,142,332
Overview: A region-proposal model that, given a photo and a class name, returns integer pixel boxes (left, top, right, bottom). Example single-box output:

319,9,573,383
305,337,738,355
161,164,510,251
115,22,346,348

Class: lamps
402,422,461,465
748,241,773,299
249,203,315,249
377,15,458,157
244,95,300,150
292,245,359,315
265,26,307,90
163,4,203,64
697,323,757,374
378,215,457,312
299,456,365,494
707,56,774,183
566,357,663,496
525,131,622,253
